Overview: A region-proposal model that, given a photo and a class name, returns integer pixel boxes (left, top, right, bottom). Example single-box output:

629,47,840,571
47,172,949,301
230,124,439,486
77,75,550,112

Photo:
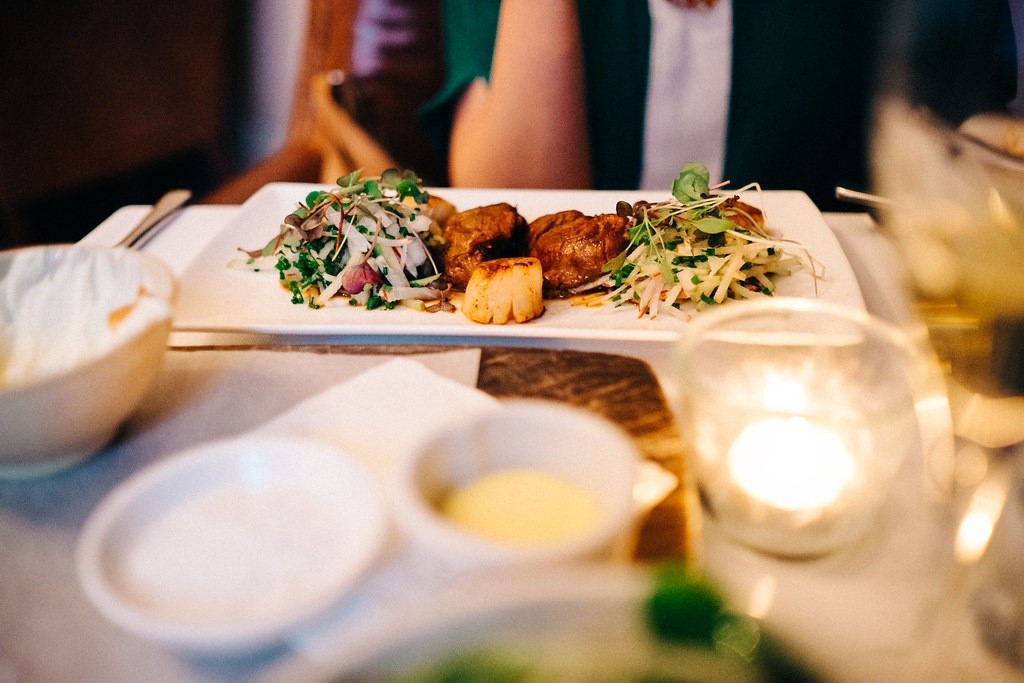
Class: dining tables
1,203,1024,683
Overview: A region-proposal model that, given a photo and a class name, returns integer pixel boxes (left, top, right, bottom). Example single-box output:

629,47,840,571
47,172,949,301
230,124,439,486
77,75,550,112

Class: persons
420,0,867,200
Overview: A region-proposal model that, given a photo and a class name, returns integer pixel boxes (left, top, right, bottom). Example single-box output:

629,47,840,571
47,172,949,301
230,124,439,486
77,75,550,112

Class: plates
168,180,870,348
76,436,389,661
228,356,678,671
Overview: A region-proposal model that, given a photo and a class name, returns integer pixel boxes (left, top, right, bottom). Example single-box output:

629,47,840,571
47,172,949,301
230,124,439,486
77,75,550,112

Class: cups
683,298,946,558
389,402,642,614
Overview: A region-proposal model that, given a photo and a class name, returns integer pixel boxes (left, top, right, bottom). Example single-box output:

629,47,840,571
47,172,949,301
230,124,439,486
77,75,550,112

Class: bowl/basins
0,247,181,484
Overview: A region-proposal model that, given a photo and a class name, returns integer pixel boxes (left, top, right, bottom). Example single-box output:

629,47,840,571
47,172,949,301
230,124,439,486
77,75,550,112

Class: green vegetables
376,558,836,683
603,162,736,284
272,167,428,310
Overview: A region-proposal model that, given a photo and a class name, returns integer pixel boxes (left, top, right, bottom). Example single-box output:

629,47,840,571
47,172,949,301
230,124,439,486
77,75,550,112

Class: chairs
194,0,357,203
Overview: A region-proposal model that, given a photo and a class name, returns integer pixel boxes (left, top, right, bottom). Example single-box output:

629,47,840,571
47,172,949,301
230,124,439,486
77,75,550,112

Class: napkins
75,200,266,349
234,363,493,677
0,344,482,683
